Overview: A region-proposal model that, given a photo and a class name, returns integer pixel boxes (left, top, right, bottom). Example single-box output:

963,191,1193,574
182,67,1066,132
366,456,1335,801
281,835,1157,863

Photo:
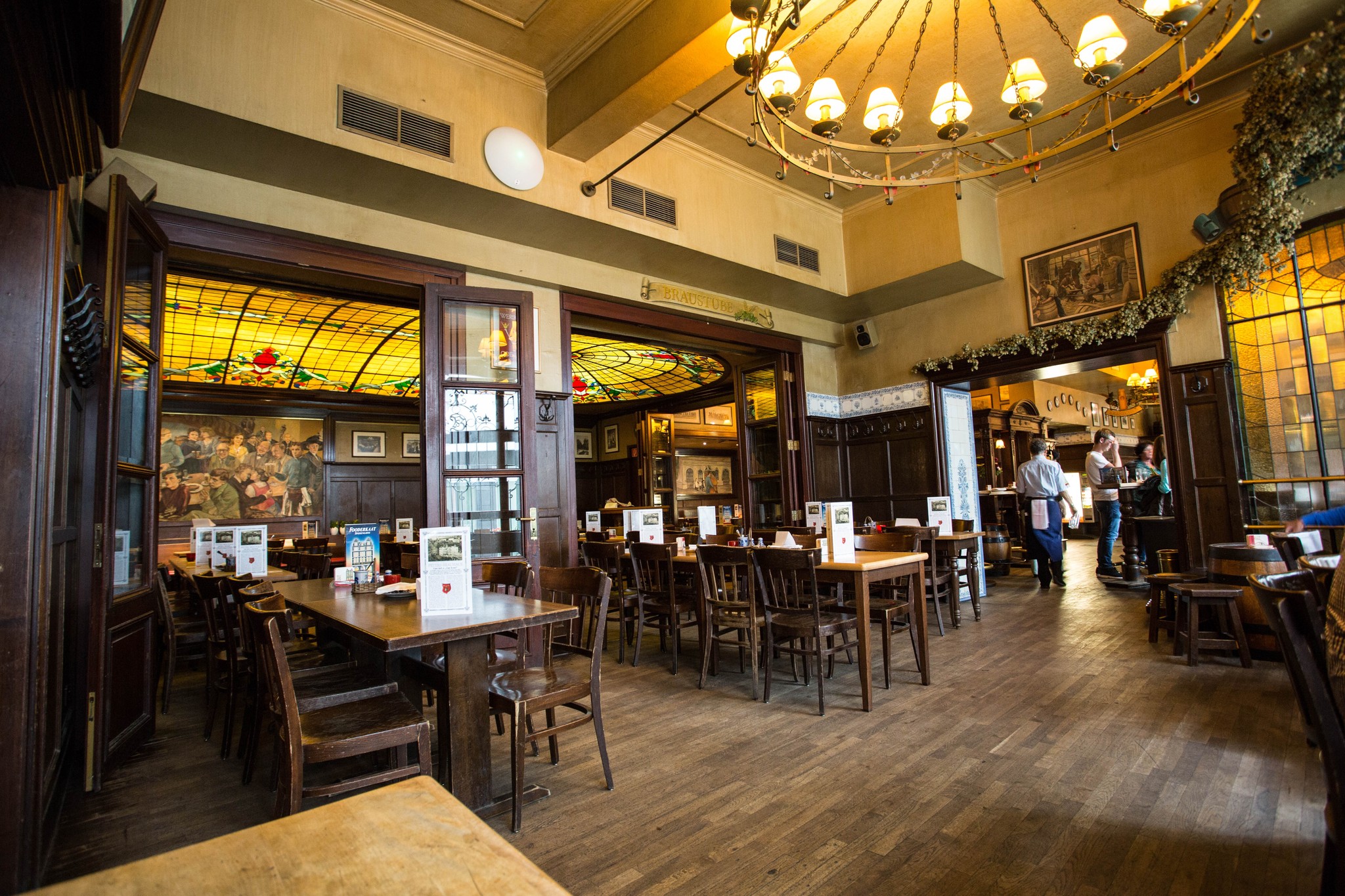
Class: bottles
385,570,392,575
864,523,871,526
1111,475,1115,480
1115,475,1119,480
748,538,756,547
757,538,764,546
370,571,382,583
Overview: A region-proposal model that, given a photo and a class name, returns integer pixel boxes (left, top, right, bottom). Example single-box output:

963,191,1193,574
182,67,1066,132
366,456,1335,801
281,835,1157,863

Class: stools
1144,573,1254,669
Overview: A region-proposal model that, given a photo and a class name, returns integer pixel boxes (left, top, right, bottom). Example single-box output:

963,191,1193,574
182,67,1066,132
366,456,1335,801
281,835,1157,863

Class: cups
738,537,748,547
728,540,738,546
186,553,196,561
354,570,368,584
226,556,236,566
869,522,876,529
383,574,401,586
1135,468,1148,482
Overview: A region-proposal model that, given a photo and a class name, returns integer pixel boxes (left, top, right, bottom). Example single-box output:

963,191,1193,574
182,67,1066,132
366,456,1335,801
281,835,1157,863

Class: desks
930,532,986,629
268,543,336,569
20,776,574,896
273,578,581,819
1094,483,1149,587
169,557,298,610
622,551,930,713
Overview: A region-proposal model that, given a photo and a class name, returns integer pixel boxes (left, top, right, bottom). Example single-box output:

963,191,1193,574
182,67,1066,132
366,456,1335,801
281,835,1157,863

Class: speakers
853,320,879,350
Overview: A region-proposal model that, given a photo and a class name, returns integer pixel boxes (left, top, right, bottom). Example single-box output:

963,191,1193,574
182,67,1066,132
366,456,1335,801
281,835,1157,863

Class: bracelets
1069,503,1074,507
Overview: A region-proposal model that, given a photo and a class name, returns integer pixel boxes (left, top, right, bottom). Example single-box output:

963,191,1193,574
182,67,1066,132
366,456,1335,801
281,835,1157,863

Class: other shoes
1052,576,1066,586
1099,567,1123,579
1040,586,1050,591
1119,554,1125,560
1096,567,1100,577
1138,561,1147,568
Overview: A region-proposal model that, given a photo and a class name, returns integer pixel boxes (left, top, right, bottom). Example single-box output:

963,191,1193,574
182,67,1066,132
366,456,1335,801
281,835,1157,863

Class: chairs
156,519,976,835
1248,555,1345,896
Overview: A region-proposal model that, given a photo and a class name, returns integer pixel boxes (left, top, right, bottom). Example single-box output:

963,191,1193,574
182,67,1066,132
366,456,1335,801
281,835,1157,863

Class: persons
1142,435,1175,517
1120,440,1162,568
1323,533,1345,732
1085,427,1128,580
1015,438,1079,590
1285,505,1345,533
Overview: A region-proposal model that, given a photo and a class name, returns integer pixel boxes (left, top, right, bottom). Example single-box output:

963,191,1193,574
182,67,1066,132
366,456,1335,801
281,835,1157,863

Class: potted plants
340,520,349,534
330,521,340,535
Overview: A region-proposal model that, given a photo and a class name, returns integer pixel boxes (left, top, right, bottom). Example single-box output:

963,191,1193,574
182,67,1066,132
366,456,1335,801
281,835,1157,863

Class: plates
383,588,417,598
1048,393,1087,417
991,489,1007,491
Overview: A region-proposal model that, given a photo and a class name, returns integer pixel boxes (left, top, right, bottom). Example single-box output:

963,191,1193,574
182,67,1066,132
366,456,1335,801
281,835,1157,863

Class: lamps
726,0,1271,203
1127,369,1160,406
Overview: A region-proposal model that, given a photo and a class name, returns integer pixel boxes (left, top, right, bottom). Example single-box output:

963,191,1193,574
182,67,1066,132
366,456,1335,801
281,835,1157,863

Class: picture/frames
574,431,593,460
604,423,620,454
402,432,421,458
1091,402,1135,429
352,430,386,458
1021,222,1147,331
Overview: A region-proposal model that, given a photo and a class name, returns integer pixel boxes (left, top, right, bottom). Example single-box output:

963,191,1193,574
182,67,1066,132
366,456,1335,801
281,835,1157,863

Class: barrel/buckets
981,523,1012,576
1207,543,1288,651
